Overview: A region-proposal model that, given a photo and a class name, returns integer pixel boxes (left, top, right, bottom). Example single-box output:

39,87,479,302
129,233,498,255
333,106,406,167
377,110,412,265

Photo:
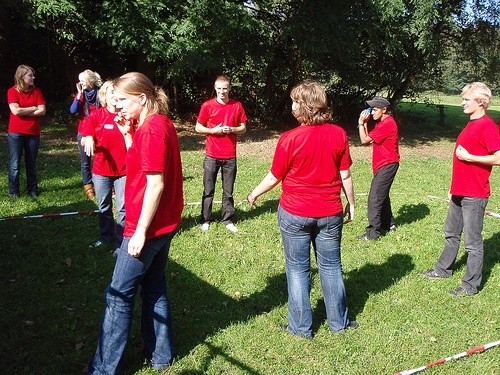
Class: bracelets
123,131,130,137
249,194,255,201
357,124,364,128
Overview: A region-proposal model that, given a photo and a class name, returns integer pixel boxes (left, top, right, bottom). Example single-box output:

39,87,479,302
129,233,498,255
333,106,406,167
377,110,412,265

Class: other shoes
226,224,237,232
347,322,359,328
89,241,103,247
84,184,94,199
381,225,396,232
418,270,439,279
283,327,287,330
113,248,120,256
358,233,368,240
449,287,467,295
202,224,209,230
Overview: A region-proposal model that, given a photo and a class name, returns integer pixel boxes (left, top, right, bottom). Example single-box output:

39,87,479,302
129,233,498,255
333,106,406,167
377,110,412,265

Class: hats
365,97,390,107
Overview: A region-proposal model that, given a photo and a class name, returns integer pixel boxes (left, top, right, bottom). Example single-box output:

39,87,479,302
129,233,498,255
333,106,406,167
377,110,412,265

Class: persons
7,64,46,200
70,69,104,199
248,82,355,340
195,75,248,233
79,81,137,257
89,72,184,375
423,82,500,296
357,97,400,240
